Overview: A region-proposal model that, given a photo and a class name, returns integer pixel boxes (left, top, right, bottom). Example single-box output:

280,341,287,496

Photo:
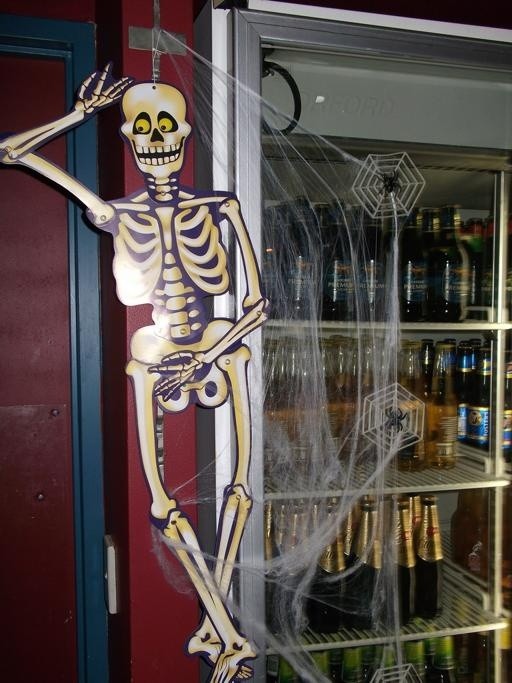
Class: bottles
268,626,510,683
263,493,445,635
262,335,512,477
263,193,512,322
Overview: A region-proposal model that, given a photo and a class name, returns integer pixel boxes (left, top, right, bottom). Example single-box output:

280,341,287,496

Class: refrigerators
197,2,512,683
